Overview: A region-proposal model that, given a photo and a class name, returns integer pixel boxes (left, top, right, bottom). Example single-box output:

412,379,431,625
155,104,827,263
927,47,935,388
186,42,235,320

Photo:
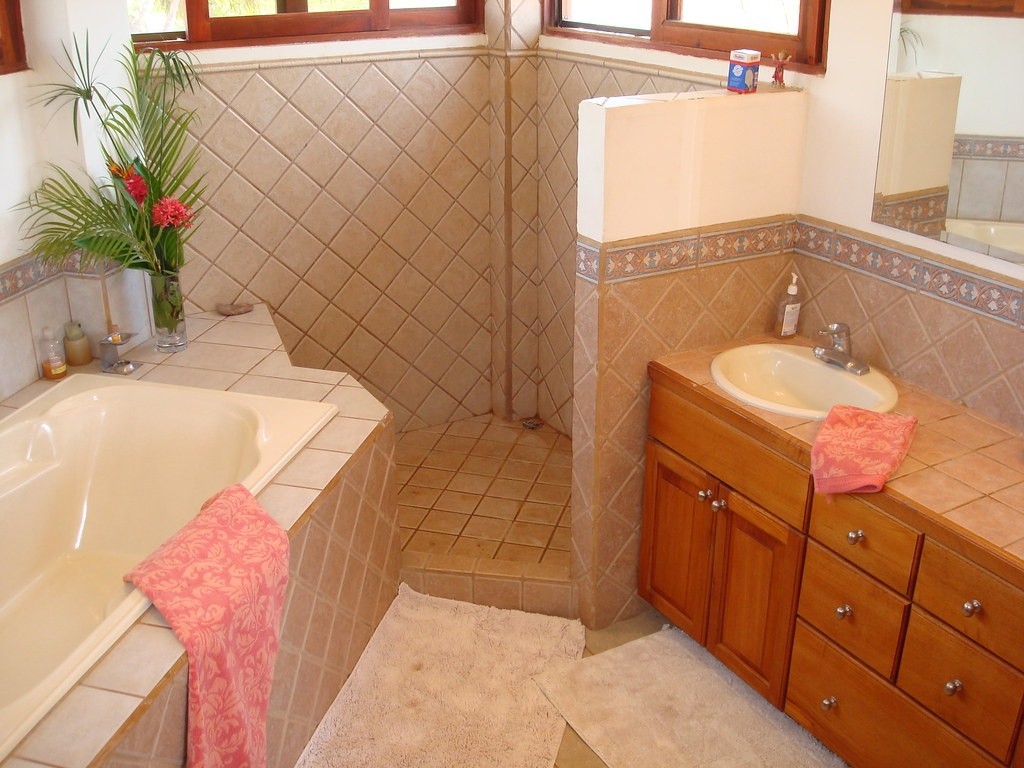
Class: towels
122,482,290,767
808,404,919,495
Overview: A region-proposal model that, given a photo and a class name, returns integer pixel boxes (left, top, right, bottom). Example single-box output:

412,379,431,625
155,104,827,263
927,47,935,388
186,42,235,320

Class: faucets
812,322,871,376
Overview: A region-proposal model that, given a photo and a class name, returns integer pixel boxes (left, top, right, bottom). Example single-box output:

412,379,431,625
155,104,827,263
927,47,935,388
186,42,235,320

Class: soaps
215,301,255,316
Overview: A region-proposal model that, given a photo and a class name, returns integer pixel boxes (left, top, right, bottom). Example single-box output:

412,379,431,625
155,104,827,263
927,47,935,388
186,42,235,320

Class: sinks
709,343,899,422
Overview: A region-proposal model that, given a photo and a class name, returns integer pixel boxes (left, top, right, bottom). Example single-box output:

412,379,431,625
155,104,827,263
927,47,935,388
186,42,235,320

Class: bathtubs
0,371,342,768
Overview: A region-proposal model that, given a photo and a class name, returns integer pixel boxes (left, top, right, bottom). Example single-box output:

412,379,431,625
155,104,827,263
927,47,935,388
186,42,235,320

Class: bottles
773,271,801,339
63,321,93,366
38,327,67,380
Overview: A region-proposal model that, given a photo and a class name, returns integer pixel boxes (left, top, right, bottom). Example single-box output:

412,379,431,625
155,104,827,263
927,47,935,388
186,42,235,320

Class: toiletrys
772,272,803,339
726,48,762,94
37,325,68,380
63,322,93,367
